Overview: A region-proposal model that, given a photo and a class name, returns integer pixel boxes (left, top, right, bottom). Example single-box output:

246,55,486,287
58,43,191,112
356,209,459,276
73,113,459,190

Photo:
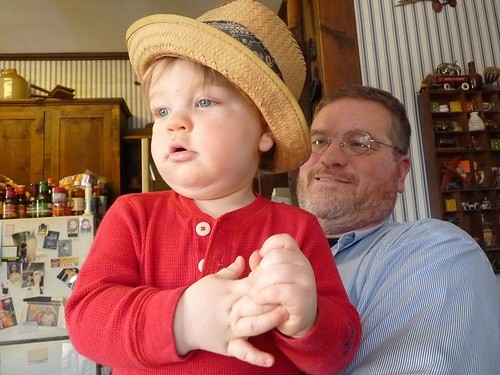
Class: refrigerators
0,215,100,375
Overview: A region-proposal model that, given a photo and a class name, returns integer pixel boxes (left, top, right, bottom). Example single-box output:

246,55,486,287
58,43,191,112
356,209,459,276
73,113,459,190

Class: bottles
468,112,485,131
0,178,85,219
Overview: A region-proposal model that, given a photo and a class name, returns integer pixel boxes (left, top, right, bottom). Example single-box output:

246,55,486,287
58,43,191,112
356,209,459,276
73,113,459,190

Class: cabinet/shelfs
416,86,500,275
0,98,133,214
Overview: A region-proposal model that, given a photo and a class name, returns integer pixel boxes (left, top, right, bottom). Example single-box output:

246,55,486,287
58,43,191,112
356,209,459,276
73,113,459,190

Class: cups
483,229,492,247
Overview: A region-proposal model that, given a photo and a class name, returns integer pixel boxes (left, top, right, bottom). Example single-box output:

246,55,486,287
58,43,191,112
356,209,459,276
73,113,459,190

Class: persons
65,0,364,375
296,82,500,375
0,218,91,331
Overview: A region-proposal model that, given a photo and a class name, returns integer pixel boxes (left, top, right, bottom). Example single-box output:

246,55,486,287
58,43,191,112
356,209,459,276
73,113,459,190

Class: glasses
310,131,405,156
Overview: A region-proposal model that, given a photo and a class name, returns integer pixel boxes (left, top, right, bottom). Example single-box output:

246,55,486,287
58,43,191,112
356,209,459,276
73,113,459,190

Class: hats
126,0,311,174
